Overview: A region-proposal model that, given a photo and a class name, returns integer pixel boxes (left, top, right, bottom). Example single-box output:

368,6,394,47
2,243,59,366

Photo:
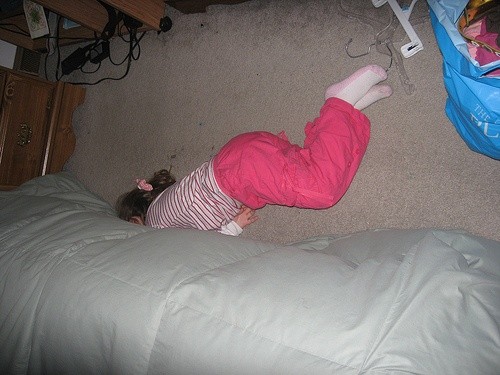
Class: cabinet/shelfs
0,0,166,55
0,65,87,192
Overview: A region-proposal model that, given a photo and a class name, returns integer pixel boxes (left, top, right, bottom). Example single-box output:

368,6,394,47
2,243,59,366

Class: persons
116,64,391,238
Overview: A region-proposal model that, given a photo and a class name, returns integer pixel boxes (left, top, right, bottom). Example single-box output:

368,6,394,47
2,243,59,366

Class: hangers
334,0,424,94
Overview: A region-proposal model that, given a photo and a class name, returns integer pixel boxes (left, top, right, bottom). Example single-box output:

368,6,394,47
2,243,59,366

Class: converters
61,40,110,74
